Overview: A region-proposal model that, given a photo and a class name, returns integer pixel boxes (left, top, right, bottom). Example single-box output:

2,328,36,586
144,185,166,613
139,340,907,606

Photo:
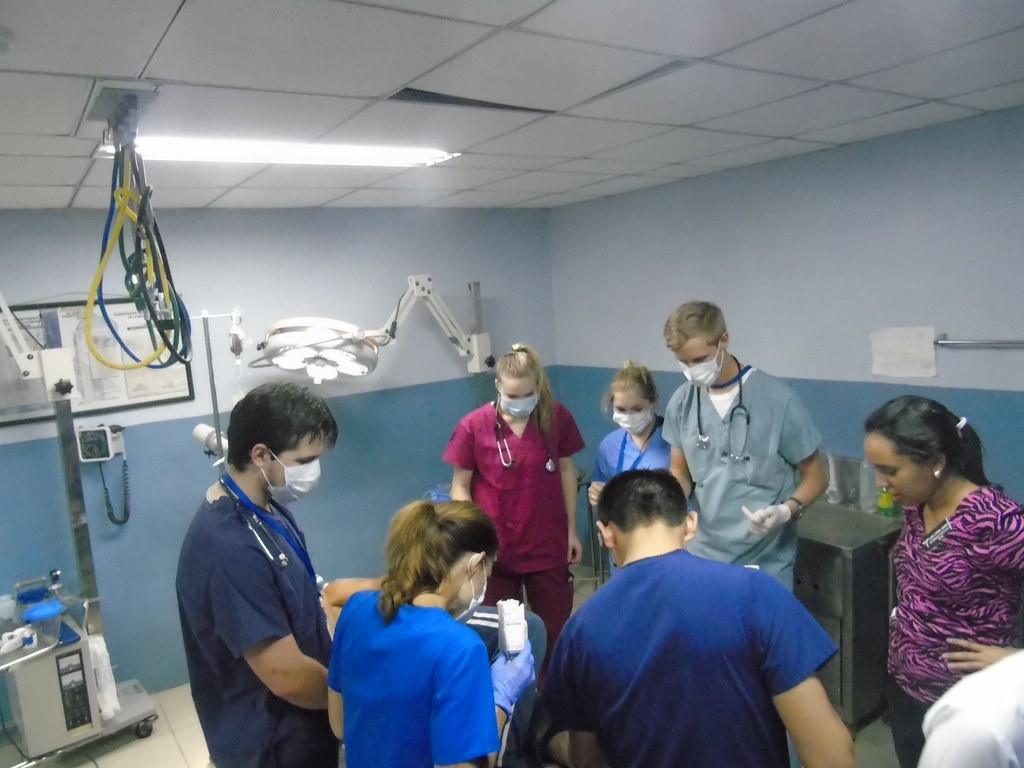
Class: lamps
245,270,496,384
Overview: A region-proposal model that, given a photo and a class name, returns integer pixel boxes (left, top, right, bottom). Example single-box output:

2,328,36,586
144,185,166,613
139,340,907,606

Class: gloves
741,504,791,535
489,639,536,724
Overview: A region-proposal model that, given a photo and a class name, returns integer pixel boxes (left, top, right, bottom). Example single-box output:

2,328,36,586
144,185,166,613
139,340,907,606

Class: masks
260,449,321,504
613,403,652,434
454,553,487,623
500,393,537,417
677,340,724,386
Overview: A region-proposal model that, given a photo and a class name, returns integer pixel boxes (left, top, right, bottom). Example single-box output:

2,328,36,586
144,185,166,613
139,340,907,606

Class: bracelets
790,497,806,522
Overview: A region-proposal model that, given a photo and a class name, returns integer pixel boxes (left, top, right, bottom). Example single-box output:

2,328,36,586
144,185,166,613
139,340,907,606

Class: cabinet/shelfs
792,502,903,742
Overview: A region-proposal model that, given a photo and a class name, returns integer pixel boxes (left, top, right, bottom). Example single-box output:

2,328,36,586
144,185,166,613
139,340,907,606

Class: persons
442,343,585,693
661,301,830,597
587,366,671,576
175,382,338,768
327,499,536,768
543,467,854,768
863,395,1024,768
917,650,1024,768
